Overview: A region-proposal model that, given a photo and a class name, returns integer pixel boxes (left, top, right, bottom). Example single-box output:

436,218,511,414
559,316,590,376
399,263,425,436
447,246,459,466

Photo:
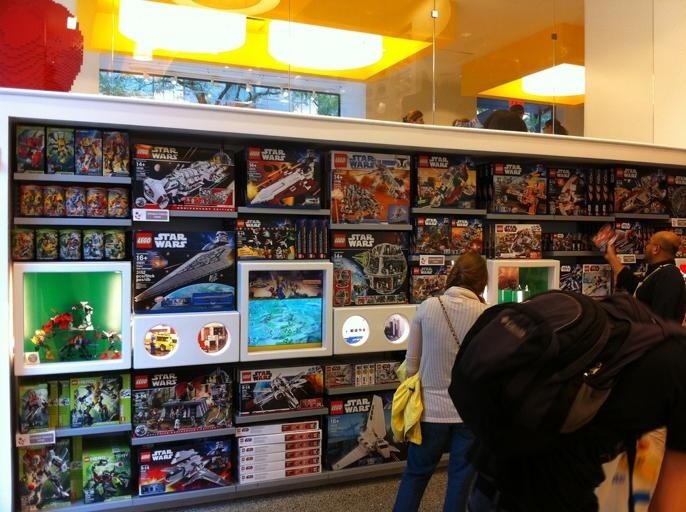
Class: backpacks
447,289,685,437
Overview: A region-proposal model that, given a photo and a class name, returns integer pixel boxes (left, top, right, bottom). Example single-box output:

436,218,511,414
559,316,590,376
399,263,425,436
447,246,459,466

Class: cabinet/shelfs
0,86,686,512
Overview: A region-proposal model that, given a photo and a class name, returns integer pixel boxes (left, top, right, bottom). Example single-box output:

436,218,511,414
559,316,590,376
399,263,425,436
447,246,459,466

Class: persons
402,103,567,135
396,232,684,512
